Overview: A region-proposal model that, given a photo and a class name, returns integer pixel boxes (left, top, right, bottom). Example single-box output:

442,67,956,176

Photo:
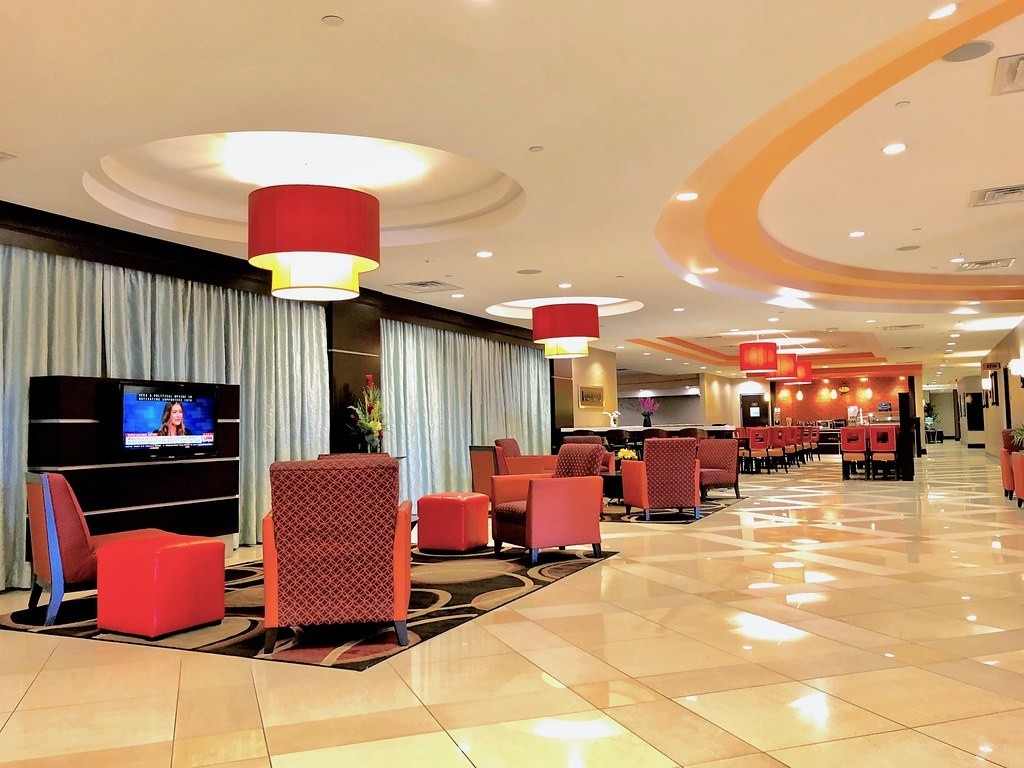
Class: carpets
0,543,620,672
597,496,747,524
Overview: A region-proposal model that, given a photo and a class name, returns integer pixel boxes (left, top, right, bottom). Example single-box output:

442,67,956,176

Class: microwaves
816,420,833,429
832,419,846,429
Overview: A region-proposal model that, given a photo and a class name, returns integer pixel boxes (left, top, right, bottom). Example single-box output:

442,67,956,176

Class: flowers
613,448,638,460
639,395,660,414
348,375,392,454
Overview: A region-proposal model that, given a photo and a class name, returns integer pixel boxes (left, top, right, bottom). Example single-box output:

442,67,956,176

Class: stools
95,537,226,637
417,492,489,551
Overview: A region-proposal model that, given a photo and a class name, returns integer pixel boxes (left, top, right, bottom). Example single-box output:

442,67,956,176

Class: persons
155,401,192,435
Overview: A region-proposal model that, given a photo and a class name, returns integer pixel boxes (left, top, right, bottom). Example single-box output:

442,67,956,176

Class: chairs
1001,426,1024,508
25,471,164,627
262,458,414,652
468,426,821,566
841,424,899,479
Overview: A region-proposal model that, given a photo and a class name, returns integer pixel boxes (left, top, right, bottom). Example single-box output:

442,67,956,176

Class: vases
643,415,651,427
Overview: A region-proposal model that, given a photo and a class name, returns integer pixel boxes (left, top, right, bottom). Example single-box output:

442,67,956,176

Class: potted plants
924,403,940,424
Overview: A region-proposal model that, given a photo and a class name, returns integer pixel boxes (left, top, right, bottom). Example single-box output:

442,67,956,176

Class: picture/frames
578,384,605,408
983,372,999,408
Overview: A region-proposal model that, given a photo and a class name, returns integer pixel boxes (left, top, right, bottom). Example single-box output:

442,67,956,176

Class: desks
561,426,736,432
926,431,944,442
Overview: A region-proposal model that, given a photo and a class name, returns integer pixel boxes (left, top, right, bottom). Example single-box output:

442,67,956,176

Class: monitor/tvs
118,381,219,460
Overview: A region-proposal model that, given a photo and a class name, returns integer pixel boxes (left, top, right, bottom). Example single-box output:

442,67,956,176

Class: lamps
739,333,872,400
247,185,381,301
532,303,600,359
